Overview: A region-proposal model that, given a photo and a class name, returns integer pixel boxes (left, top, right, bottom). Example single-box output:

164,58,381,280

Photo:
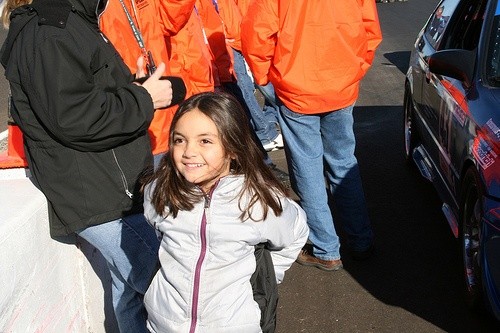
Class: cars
404,0,500,333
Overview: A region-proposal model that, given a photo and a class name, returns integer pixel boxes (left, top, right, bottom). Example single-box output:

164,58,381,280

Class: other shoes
262,133,284,149
269,164,290,183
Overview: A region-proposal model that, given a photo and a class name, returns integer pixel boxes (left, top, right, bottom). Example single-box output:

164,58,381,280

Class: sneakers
340,246,371,261
295,243,342,271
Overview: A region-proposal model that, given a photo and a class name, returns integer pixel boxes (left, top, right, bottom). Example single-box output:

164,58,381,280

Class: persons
0,0,172,333
239,0,383,272
143,92,310,333
98,0,290,181
